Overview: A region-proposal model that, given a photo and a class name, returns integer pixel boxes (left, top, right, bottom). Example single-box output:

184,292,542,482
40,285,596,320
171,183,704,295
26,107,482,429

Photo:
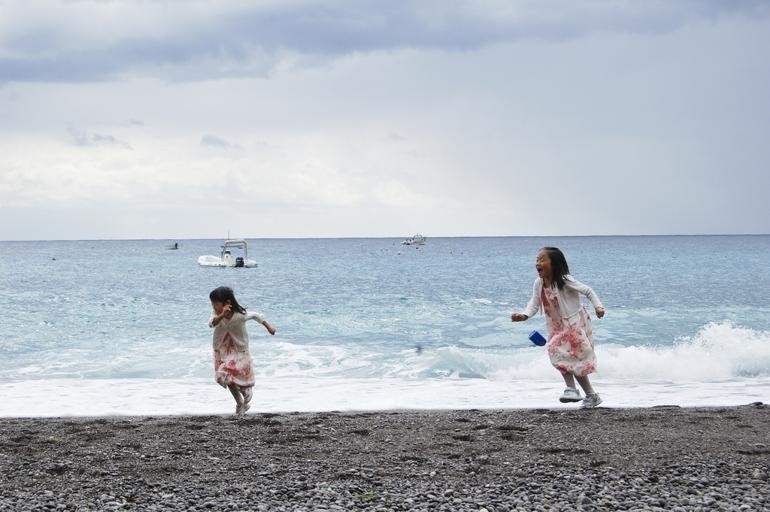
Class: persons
208,286,276,414
511,247,605,409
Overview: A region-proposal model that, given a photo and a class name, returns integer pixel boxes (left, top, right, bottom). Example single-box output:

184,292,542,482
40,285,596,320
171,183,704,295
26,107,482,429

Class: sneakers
559,387,583,403
235,402,251,417
242,386,253,404
579,392,603,410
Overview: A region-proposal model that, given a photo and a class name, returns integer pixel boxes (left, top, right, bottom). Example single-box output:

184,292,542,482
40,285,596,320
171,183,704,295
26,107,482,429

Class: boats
404,234,426,245
197,240,258,268
